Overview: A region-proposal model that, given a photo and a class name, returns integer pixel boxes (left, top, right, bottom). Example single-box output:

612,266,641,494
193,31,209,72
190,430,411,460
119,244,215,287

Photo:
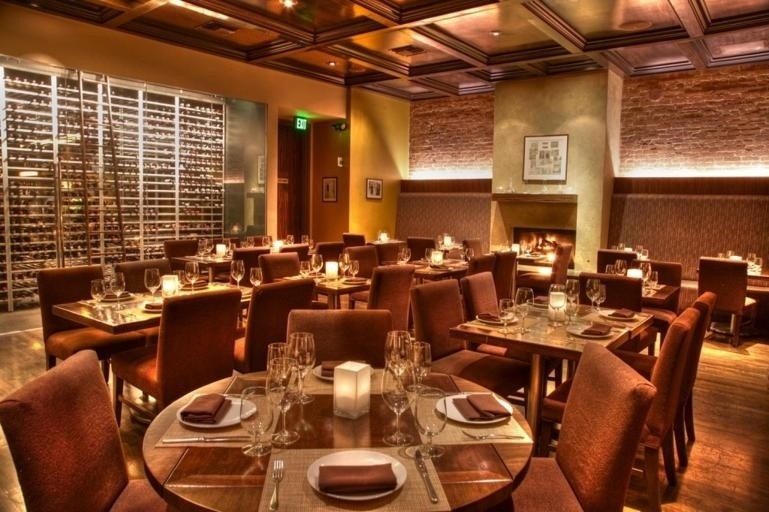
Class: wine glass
381,330,447,458
240,330,317,458
398,246,474,271
747,252,763,274
90,234,360,311
499,278,606,333
606,242,657,295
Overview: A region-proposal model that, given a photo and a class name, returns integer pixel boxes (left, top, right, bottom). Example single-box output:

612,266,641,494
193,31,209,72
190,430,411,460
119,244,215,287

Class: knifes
415,450,438,503
162,436,252,444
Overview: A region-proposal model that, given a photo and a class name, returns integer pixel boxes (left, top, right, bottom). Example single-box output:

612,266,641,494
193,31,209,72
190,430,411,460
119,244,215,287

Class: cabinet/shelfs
224,183,244,232
247,192,265,225
0,54,229,312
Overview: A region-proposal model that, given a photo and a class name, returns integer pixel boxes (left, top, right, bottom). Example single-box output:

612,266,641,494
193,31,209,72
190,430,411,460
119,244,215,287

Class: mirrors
223,99,268,241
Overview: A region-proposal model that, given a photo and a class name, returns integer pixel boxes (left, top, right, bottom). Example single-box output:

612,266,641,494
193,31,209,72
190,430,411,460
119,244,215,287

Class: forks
270,459,284,511
462,429,524,441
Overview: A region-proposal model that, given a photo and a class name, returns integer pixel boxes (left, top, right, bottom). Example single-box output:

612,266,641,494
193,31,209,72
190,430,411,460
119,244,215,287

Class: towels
319,463,397,493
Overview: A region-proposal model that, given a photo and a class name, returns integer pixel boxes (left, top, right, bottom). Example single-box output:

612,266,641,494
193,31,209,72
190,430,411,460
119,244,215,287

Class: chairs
539,307,700,512
511,343,657,512
578,272,642,312
597,249,637,273
36,232,574,427
0,349,180,512
698,256,757,348
612,291,717,467
633,260,684,315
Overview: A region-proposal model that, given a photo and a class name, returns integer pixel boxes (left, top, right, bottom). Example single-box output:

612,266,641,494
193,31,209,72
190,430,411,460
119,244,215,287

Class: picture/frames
522,134,568,182
366,178,383,200
322,177,337,202
257,154,265,185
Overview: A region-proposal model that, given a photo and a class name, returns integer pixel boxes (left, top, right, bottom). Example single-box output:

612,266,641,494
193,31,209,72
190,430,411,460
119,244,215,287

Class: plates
567,310,638,339
342,281,367,285
437,394,513,425
308,450,407,501
177,397,256,429
314,364,376,380
475,311,518,323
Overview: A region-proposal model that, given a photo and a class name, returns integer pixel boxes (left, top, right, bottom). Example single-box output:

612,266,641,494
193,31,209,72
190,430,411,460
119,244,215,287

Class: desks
142,364,534,512
448,294,662,461
